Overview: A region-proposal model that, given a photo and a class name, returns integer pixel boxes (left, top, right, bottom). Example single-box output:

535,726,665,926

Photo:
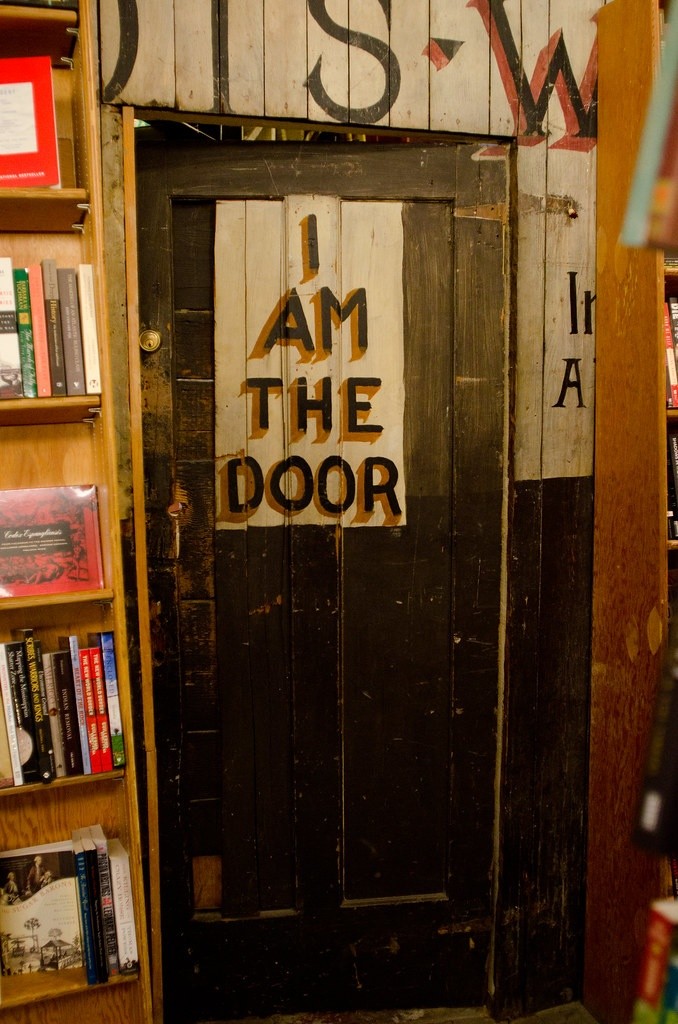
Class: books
0,823,142,988
0,626,126,788
663,296,678,410
0,256,100,400
629,648,678,1024
658,8,673,78
1,57,63,191
0,488,104,596
667,436,678,540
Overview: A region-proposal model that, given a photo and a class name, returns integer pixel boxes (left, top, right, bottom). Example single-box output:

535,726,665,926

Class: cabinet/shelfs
580,1,678,1024
0,1,153,1024
